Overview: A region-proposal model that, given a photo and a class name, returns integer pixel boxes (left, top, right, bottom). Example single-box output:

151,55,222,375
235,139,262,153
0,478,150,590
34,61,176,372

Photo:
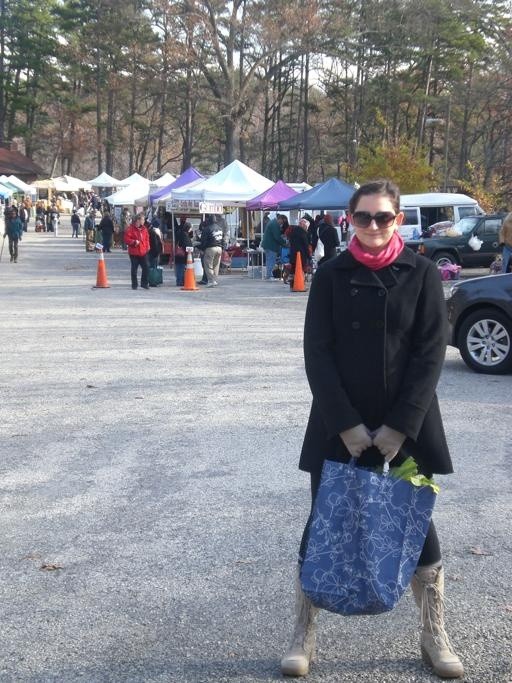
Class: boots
279,564,320,677
410,566,464,679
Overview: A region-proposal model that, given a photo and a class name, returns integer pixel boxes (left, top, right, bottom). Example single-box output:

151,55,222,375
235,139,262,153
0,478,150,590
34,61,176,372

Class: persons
255,211,340,282
499,211,512,273
280,178,463,677
3,190,230,290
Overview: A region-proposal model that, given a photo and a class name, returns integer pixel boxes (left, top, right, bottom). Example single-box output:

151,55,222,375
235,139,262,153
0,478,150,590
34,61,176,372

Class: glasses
349,211,398,229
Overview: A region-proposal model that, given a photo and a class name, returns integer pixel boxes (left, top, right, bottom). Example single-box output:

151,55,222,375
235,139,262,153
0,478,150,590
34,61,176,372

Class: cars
444,272,512,379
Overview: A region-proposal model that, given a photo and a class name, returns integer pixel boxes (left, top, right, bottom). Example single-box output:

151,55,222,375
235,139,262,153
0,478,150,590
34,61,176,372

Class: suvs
403,213,507,273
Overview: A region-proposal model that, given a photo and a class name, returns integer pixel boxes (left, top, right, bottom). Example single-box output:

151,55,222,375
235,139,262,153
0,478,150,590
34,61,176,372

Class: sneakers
197,280,218,288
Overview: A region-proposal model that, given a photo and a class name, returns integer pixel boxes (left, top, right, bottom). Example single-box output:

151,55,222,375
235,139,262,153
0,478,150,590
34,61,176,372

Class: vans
394,192,488,244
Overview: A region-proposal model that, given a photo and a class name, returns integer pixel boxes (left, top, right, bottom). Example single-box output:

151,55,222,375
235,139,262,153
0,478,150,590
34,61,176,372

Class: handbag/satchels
147,263,163,286
299,434,437,617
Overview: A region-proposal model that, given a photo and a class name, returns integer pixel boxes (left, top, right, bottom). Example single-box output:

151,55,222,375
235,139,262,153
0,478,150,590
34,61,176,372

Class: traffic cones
181,252,199,291
94,249,112,289
291,251,308,293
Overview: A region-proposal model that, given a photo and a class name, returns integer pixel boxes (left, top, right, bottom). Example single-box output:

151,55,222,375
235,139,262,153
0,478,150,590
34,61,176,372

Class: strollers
35,215,46,232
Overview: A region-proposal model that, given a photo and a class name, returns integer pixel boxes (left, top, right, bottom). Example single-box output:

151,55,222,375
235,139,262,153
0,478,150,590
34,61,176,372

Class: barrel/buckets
193,258,204,283
